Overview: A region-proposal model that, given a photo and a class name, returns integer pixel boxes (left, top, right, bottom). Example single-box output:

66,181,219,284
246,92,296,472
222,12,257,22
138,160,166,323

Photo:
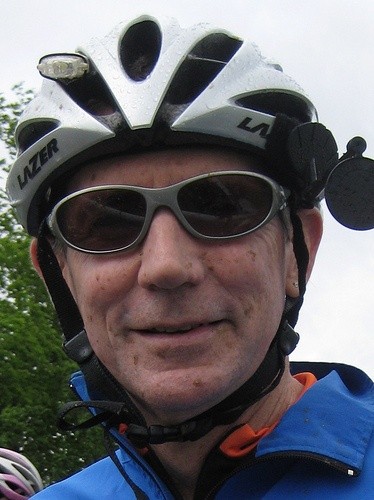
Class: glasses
44,170,289,257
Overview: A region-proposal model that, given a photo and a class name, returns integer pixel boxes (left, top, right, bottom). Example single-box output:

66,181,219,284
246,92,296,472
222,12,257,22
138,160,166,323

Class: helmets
5,12,374,233
0,446,44,500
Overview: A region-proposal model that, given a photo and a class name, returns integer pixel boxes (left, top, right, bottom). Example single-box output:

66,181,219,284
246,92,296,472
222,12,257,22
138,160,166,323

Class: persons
0,9,374,500
